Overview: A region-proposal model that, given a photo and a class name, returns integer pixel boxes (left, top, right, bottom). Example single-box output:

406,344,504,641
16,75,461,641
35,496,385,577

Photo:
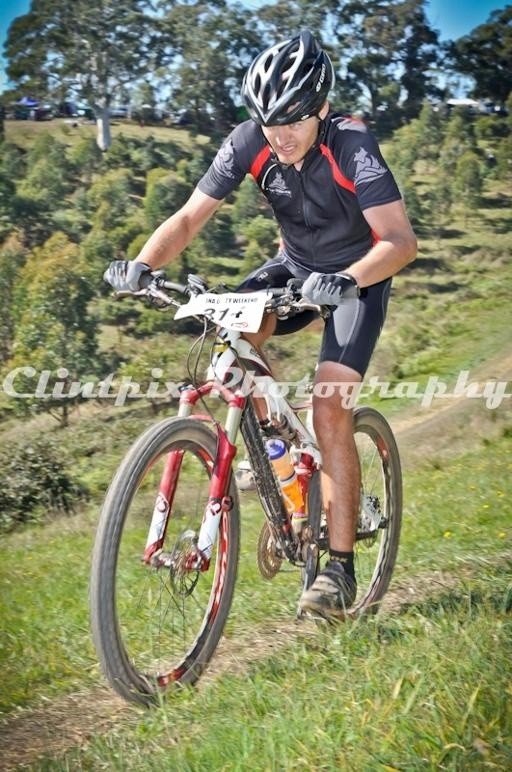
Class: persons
102,29,418,620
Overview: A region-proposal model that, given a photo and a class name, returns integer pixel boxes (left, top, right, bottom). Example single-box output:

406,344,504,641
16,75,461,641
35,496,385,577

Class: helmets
240,29,339,130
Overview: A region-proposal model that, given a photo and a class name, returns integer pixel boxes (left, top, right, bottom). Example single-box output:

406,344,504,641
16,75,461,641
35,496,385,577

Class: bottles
264,436,302,512
293,448,316,520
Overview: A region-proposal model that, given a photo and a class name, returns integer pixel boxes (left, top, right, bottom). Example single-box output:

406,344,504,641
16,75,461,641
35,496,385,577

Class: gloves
99,255,156,294
300,266,365,310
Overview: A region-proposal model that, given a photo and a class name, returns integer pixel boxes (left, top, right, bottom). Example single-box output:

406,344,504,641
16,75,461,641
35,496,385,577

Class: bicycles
84,261,408,707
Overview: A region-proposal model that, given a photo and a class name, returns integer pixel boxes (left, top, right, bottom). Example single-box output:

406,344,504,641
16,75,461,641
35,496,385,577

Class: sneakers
234,447,262,496
298,557,362,614
